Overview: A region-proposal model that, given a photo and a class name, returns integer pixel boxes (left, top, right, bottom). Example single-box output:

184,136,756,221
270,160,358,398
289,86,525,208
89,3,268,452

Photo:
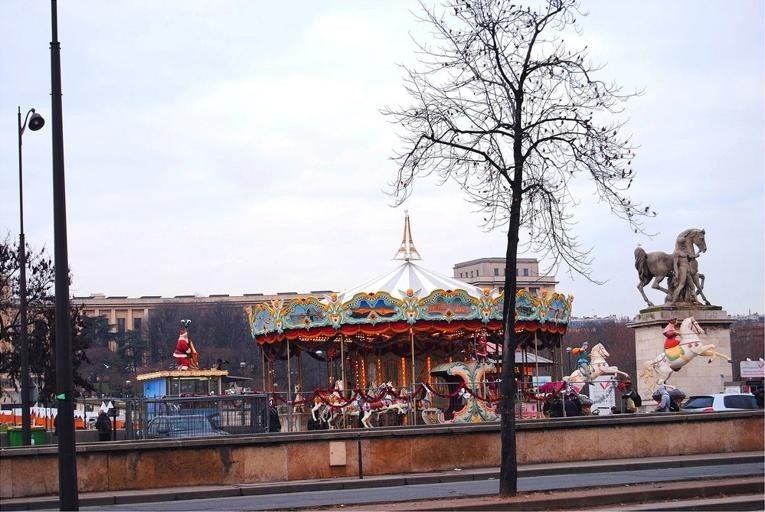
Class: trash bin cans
7,426,45,449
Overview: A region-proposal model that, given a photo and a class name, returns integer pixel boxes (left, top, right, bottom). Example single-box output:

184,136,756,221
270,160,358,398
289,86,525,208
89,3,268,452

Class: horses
634,228,711,306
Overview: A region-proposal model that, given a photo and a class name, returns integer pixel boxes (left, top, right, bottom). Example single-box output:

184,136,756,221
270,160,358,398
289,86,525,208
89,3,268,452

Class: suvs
680,393,762,413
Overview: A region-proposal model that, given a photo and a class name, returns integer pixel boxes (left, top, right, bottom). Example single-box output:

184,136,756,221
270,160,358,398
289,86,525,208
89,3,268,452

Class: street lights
565,347,573,376
103,364,113,399
316,349,322,389
240,362,247,386
17,106,46,447
124,364,138,398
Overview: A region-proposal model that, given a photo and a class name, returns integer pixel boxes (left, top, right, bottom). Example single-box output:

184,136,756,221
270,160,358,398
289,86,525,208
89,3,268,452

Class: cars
136,415,231,439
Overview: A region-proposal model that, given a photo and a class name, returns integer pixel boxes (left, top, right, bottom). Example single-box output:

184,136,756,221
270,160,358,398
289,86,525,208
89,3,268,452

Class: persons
172,329,191,371
54,414,58,436
576,342,593,378
542,393,582,417
662,316,681,349
95,409,112,441
